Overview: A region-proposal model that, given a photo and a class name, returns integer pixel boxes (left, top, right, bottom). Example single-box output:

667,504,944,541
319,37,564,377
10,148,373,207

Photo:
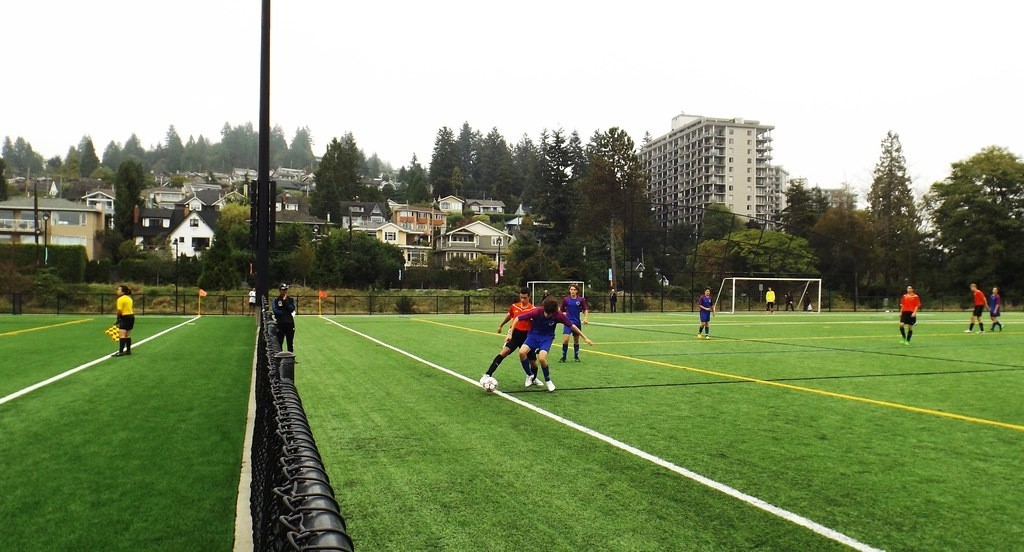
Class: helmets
279,282,289,290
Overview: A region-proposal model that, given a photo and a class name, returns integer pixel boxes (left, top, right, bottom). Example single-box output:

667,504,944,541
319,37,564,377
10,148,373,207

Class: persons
609,289,617,314
540,290,549,305
988,286,1003,332
898,284,920,345
505,295,593,392
765,287,775,315
964,283,990,334
116,285,135,356
557,284,588,363
804,292,811,311
697,286,716,339
246,287,256,316
784,289,795,312
271,283,300,364
479,287,544,386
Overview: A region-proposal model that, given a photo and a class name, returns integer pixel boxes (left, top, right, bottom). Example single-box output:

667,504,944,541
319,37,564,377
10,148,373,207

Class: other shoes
559,357,567,363
545,379,555,391
533,377,544,386
525,374,534,387
698,334,702,339
574,358,581,362
111,352,124,356
123,351,131,355
905,340,911,345
976,331,984,333
900,336,906,344
706,335,711,340
480,373,492,385
999,324,1003,331
964,328,973,332
987,329,994,332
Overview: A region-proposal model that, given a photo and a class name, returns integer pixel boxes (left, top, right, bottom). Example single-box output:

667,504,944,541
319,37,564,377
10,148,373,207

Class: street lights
497,237,502,282
172,238,178,313
43,212,50,265
35,228,41,267
312,225,319,248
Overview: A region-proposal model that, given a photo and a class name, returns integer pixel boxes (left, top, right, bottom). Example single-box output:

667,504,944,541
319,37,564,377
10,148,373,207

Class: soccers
483,377,498,392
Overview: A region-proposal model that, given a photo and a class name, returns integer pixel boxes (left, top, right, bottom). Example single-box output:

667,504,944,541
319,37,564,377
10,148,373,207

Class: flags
104,323,120,343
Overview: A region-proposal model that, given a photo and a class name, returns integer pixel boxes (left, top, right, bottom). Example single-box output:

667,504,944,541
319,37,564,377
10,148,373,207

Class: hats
611,289,615,292
